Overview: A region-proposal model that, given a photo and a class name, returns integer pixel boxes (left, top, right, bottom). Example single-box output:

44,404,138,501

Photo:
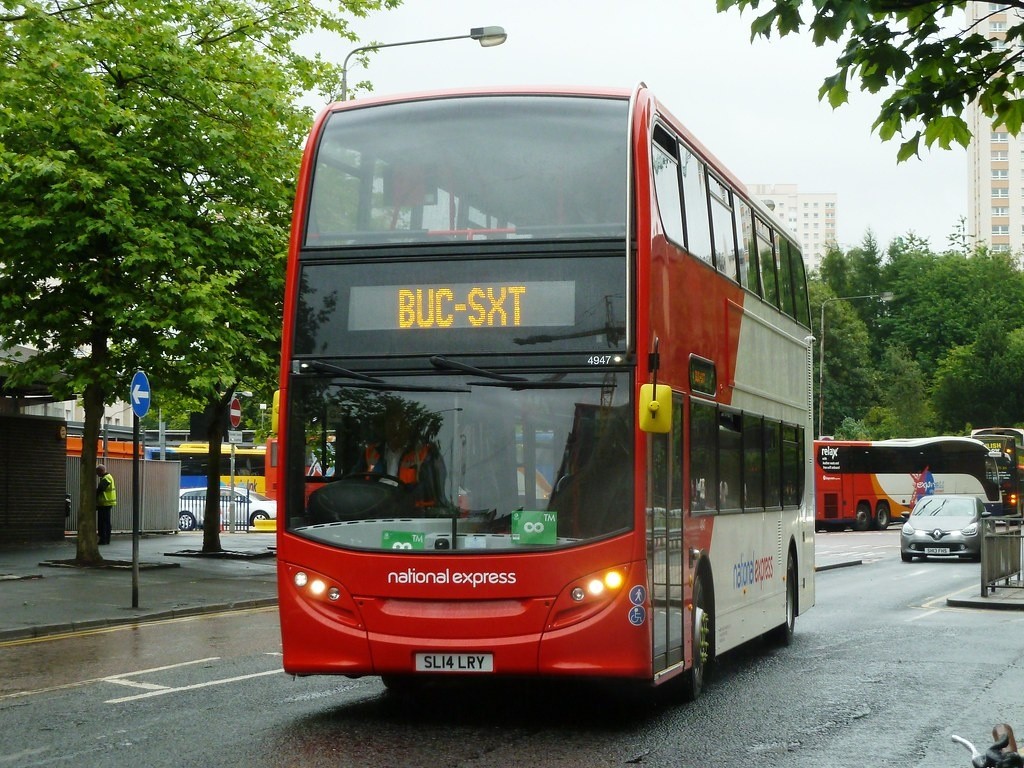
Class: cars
900,494,996,562
179,485,277,532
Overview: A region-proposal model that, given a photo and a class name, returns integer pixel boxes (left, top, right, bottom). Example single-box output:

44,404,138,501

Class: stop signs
230,398,242,428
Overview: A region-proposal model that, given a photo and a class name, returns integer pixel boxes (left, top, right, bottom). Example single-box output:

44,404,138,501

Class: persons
343,397,448,513
96,463,118,549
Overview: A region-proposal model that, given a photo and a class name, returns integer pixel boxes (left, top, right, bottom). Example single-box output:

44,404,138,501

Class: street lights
229,384,254,535
341,25,511,101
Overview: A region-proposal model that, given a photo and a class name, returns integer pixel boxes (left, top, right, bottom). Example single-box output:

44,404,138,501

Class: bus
815,436,1012,532
264,429,332,518
143,443,266,496
971,428,1024,526
275,84,814,706
509,428,561,515
